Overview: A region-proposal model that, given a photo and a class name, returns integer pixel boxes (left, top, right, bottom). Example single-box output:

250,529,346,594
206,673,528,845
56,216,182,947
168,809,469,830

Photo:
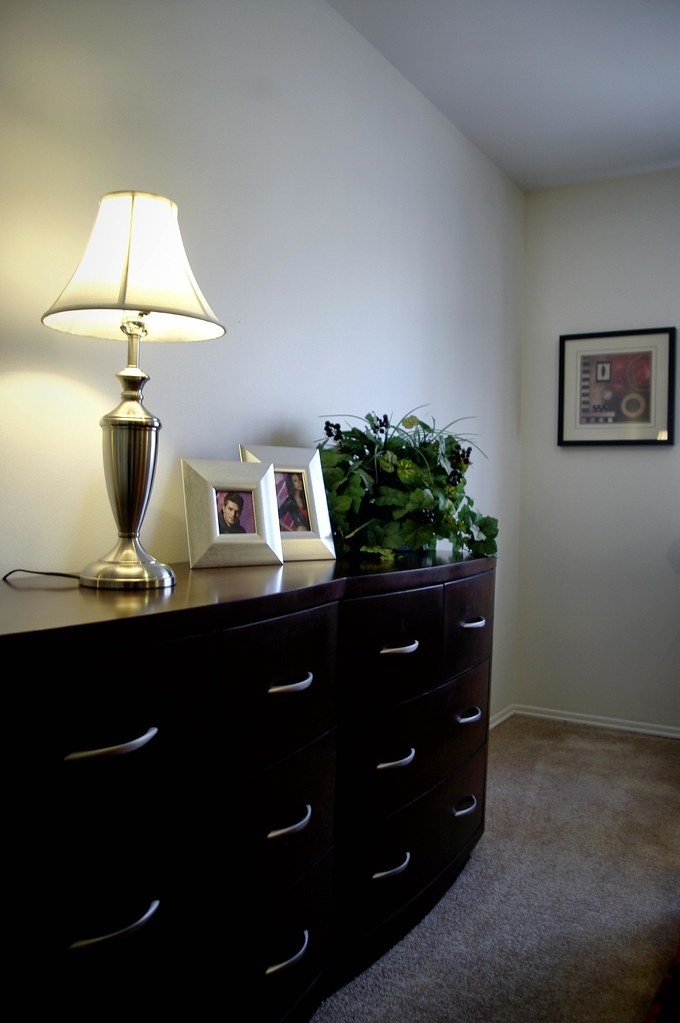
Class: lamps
41,191,228,591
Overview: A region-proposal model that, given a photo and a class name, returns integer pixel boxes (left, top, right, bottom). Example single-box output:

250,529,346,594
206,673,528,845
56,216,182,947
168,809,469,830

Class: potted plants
307,403,501,569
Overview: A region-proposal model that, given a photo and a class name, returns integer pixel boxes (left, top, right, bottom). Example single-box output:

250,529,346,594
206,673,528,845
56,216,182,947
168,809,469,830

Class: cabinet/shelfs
0,543,506,1023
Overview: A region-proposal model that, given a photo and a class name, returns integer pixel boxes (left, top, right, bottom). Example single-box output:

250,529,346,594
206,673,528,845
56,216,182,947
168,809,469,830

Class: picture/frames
179,459,283,568
558,328,676,445
239,445,338,560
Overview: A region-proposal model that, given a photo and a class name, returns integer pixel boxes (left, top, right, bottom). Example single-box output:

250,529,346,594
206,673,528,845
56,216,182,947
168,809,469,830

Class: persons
278,473,311,531
218,492,247,534
